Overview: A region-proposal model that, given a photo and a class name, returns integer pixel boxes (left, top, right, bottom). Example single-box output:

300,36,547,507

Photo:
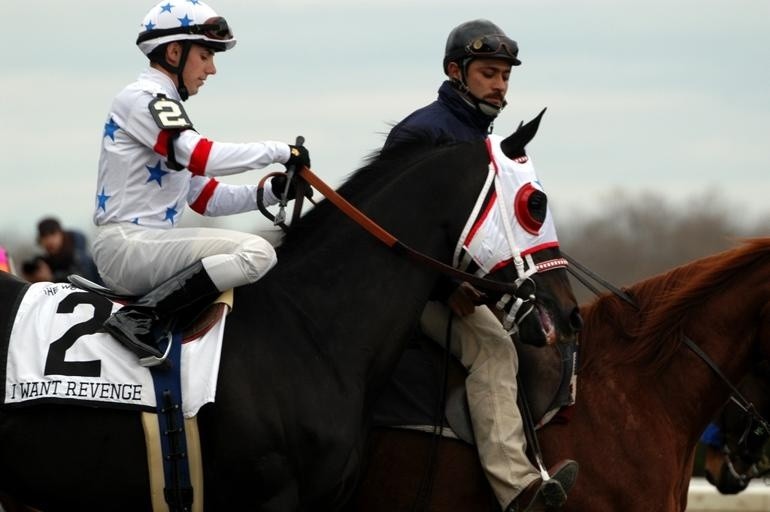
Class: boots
101,257,225,375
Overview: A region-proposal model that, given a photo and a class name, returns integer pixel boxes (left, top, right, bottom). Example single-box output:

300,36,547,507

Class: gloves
282,143,310,172
270,171,314,201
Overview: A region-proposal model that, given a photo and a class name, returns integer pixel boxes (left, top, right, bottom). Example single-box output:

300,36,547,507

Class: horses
0,104,584,512
432,233,769,512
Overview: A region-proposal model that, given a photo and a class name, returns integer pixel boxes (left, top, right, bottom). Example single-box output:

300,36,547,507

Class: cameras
22,255,46,276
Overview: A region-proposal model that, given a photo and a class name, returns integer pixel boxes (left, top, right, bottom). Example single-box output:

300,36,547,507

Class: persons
25,217,108,290
86,1,314,364
380,18,582,508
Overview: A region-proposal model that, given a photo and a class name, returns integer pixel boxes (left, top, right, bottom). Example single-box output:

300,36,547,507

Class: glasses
464,32,518,59
193,16,234,40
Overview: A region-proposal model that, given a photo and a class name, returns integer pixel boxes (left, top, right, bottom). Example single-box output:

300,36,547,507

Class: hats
35,219,60,241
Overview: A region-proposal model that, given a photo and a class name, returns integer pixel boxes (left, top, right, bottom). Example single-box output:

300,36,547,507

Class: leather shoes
507,457,581,512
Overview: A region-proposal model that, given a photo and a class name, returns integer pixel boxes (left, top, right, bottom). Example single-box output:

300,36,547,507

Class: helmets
135,0,238,62
443,19,523,76
